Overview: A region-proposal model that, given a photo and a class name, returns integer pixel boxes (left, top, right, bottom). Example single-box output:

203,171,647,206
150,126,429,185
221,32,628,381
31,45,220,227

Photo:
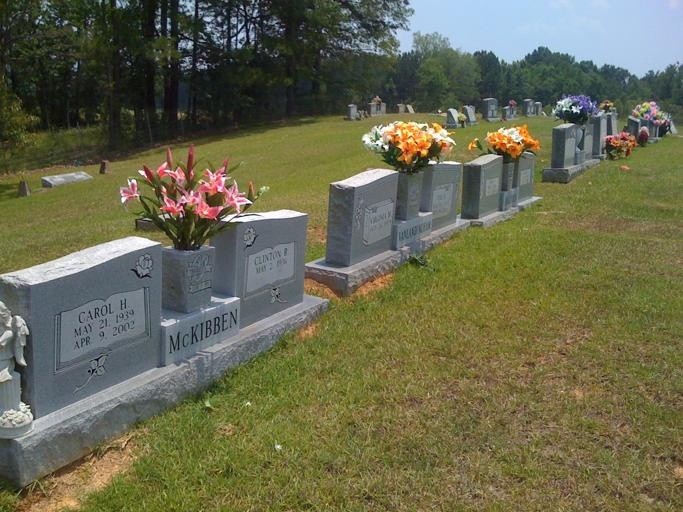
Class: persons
0,301,30,382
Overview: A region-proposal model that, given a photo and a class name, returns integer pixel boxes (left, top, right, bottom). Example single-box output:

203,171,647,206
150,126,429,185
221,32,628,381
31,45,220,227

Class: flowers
359,116,453,176
639,125,650,143
117,144,268,252
600,97,616,117
456,111,467,123
506,98,516,105
627,98,670,125
548,91,600,126
467,121,538,164
606,133,638,159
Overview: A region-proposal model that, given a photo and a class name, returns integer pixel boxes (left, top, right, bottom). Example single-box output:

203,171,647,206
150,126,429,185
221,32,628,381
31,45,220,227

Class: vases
574,124,583,154
501,162,515,193
614,148,626,160
393,171,426,221
158,243,218,317
